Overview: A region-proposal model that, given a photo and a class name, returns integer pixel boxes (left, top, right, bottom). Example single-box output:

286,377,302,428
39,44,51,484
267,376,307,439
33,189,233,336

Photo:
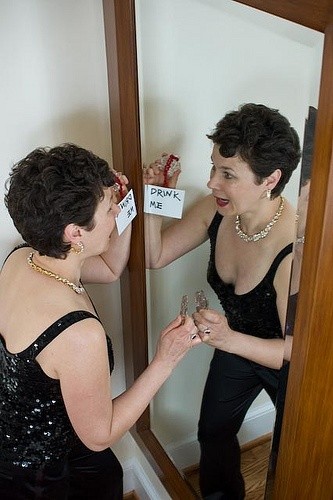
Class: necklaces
26,251,86,297
229,195,285,242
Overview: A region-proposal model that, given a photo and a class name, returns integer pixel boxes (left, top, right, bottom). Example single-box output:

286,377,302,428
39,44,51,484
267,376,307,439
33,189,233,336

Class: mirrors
101,0,332,499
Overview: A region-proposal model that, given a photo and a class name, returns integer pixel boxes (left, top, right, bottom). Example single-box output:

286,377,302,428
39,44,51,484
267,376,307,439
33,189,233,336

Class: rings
202,329,212,334
189,333,196,341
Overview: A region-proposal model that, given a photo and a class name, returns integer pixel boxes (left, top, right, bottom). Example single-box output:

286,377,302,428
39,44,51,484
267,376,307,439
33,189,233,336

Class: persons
144,102,318,500
0,142,198,500
